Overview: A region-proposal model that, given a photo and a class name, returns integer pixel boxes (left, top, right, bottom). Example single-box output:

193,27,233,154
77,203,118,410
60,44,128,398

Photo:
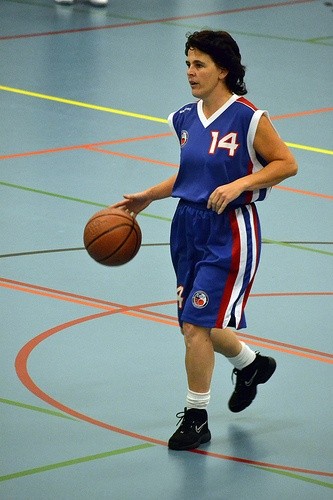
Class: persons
109,29,300,450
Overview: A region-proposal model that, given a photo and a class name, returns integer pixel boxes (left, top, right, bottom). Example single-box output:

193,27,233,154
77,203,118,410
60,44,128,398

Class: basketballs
83,209,142,266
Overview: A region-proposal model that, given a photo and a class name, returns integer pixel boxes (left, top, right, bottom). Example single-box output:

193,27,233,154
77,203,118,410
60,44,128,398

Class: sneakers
228,352,277,413
168,408,212,450
